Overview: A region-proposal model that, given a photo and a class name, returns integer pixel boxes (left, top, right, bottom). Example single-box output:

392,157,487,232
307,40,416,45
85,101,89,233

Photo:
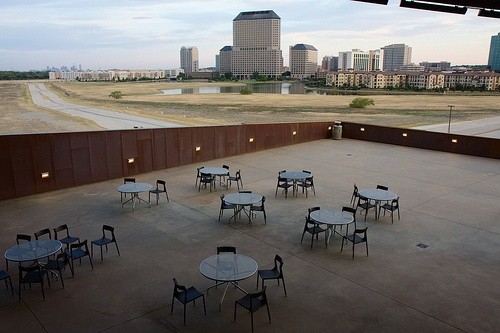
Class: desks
117,183,153,210
280,172,312,194
310,210,354,245
5,239,62,281
200,167,228,189
224,193,262,223
359,188,397,221
199,253,258,311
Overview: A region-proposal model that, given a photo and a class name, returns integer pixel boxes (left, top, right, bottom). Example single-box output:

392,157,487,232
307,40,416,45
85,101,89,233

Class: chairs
121,178,135,202
218,191,266,225
257,255,287,298
195,165,243,192
0,224,120,303
216,246,238,288
350,183,400,224
171,278,206,326
149,179,169,205
301,207,369,259
234,285,271,333
275,170,315,199
